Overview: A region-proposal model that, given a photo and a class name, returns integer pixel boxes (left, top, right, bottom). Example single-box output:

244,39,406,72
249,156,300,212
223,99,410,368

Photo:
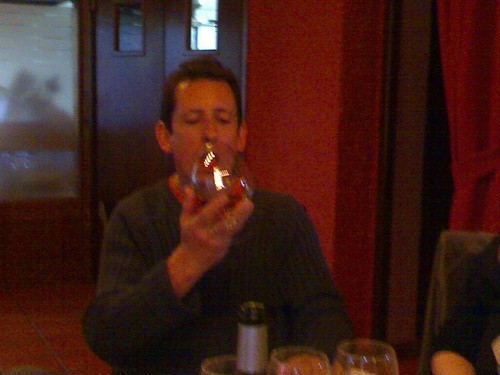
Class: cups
192,141,253,209
332,338,398,375
266,345,331,374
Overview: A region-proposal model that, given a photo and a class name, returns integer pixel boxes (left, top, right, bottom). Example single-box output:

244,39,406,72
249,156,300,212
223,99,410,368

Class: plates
201,355,284,375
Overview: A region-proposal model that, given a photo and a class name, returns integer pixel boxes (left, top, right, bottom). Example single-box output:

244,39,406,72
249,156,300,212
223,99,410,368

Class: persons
81,52,352,375
423,234,499,375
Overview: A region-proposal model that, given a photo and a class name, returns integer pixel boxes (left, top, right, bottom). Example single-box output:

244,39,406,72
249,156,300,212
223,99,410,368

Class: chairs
416,229,497,374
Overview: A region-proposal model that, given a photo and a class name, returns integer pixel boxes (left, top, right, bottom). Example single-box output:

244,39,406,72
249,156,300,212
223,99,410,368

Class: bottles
236,301,268,375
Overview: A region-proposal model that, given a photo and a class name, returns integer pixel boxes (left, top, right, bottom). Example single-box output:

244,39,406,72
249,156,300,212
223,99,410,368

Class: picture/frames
113,4,146,56
